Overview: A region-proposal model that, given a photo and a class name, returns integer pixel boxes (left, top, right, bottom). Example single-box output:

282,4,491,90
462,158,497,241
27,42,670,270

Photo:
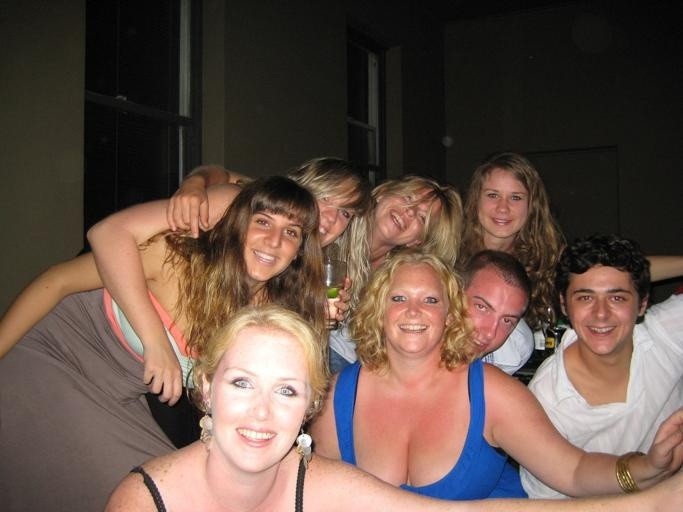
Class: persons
509,230,682,503
102,301,681,512
84,151,376,408
326,247,536,387
298,246,681,503
0,170,326,511
452,149,682,338
168,162,465,330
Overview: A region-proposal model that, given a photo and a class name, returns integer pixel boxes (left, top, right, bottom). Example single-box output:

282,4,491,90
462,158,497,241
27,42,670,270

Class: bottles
534,313,567,356
322,256,343,331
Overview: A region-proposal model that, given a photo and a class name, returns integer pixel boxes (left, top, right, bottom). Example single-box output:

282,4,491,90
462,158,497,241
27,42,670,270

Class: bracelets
614,451,647,497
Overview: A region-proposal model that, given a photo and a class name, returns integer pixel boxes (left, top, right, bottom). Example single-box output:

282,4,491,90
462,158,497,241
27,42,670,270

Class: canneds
544,326,566,353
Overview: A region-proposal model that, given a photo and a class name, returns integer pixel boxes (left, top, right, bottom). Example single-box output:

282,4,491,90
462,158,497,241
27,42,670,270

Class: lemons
326,288,339,298
